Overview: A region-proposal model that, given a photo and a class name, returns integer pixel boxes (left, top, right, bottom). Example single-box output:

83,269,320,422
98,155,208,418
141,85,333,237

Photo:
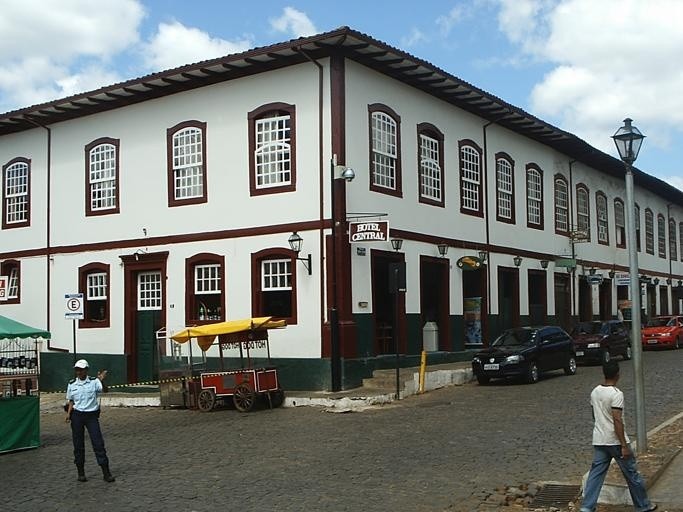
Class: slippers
638,502,659,512
579,506,597,512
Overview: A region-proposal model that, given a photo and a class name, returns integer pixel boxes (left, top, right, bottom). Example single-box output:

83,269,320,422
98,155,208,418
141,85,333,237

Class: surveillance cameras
344,167,355,182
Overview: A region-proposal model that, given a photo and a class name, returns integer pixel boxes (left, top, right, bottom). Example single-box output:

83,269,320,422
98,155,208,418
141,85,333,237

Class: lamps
478,252,573,275
390,238,403,255
437,243,449,260
288,230,311,275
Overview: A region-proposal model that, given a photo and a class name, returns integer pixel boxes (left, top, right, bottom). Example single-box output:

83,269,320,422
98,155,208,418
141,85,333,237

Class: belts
71,410,98,417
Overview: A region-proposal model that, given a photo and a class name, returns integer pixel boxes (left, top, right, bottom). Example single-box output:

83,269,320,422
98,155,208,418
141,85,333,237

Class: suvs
470,323,577,384
568,318,632,367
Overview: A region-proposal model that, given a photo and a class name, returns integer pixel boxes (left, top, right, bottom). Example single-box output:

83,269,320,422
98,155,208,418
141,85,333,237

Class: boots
103,468,115,482
77,462,86,482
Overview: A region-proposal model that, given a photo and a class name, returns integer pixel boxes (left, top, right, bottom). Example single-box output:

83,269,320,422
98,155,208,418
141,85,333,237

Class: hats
73,359,90,369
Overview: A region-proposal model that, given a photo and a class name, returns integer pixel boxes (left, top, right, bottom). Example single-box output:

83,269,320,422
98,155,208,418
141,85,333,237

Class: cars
641,315,683,352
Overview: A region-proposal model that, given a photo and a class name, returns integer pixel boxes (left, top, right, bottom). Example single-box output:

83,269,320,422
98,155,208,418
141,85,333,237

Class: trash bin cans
422,321,440,353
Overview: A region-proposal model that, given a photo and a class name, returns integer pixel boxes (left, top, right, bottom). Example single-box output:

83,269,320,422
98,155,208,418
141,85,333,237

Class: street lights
609,117,653,457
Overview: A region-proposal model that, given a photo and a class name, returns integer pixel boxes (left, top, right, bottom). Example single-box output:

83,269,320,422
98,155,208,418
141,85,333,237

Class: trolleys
170,315,290,411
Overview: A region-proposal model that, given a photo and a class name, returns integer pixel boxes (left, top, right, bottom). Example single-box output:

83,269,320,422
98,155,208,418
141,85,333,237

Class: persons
63,358,115,482
577,358,658,511
